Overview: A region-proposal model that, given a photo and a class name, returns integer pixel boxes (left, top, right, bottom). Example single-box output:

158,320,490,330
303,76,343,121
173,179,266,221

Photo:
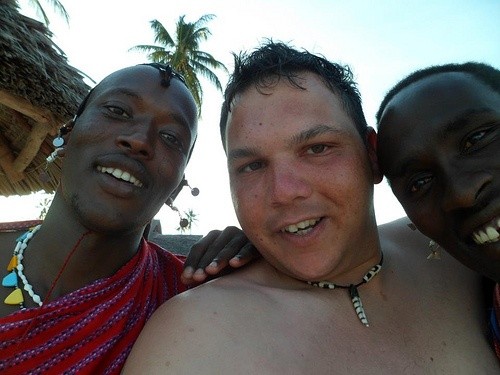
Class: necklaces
294,244,384,328
2,222,50,308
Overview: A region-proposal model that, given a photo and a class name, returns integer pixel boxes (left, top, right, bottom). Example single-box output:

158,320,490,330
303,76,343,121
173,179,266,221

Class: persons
374,60,500,368
0,60,263,375
123,38,500,375
